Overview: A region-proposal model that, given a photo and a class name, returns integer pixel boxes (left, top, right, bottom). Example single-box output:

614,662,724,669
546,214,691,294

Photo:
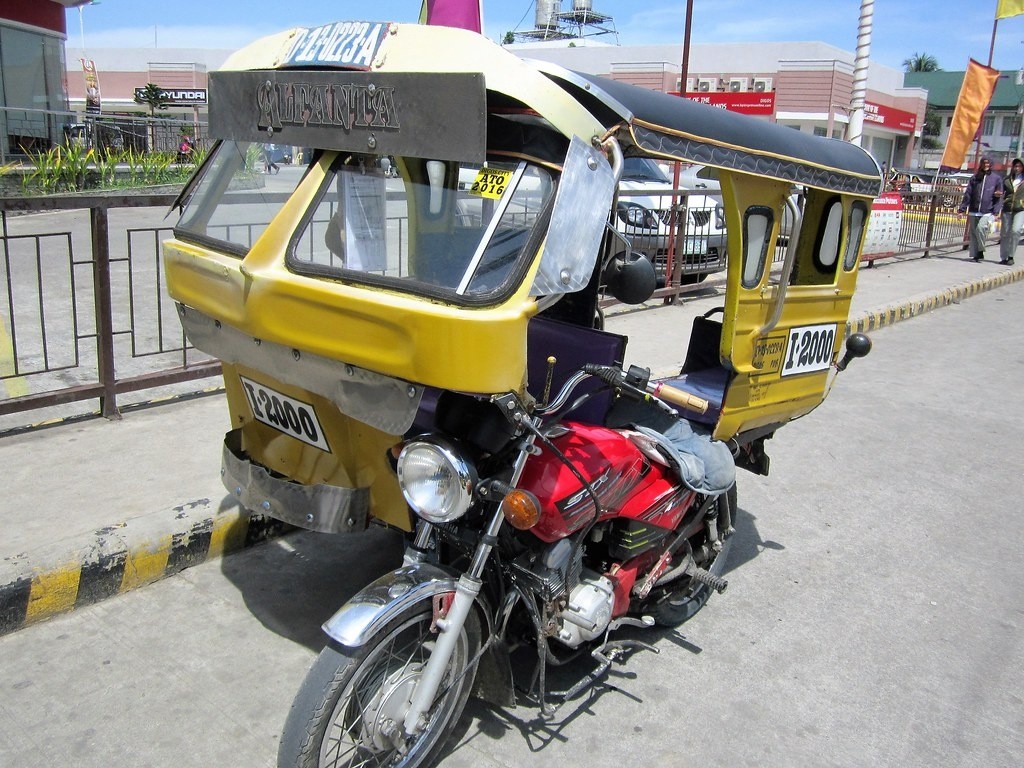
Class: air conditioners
676,78,694,92
754,78,772,92
728,77,748,92
697,78,717,92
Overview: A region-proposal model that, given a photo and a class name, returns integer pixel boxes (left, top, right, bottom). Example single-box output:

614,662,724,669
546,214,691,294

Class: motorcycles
159,21,885,768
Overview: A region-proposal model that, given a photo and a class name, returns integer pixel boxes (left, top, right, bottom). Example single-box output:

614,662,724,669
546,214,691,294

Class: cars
669,164,808,239
887,168,974,207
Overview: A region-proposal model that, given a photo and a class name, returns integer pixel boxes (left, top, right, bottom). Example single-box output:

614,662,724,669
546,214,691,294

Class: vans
452,159,727,286
72,124,124,153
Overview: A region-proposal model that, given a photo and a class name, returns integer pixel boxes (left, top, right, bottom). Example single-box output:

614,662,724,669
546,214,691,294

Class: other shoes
969,256,985,263
274,167,280,175
261,170,272,175
999,260,1015,265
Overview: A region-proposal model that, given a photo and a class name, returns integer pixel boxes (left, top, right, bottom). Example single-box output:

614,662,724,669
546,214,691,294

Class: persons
993,158,1024,266
262,142,280,174
956,156,1004,261
881,161,886,178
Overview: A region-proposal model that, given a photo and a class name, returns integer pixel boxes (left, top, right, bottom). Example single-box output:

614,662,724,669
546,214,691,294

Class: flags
941,59,1002,177
994,0,1024,20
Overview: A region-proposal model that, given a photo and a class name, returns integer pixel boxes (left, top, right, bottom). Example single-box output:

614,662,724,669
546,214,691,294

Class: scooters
175,134,200,164
